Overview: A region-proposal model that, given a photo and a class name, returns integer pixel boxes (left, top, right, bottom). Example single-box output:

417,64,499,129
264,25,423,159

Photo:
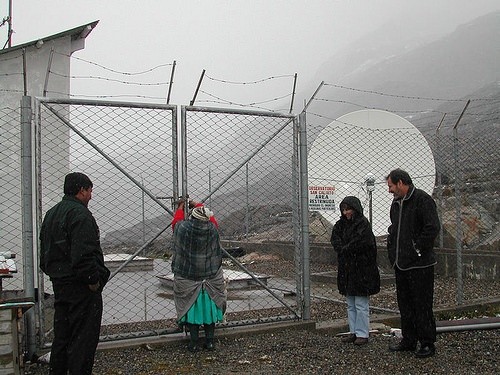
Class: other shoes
203,341,215,350
186,343,200,351
342,333,369,345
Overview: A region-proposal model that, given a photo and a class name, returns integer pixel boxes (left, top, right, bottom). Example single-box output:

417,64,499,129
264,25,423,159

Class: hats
192,206,214,221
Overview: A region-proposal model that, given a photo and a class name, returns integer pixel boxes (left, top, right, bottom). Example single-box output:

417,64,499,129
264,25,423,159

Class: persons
172,198,226,352
329,196,381,346
386,168,441,357
40,172,111,375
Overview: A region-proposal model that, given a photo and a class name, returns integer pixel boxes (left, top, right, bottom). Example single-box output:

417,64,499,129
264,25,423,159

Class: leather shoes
415,344,437,358
389,338,416,351
